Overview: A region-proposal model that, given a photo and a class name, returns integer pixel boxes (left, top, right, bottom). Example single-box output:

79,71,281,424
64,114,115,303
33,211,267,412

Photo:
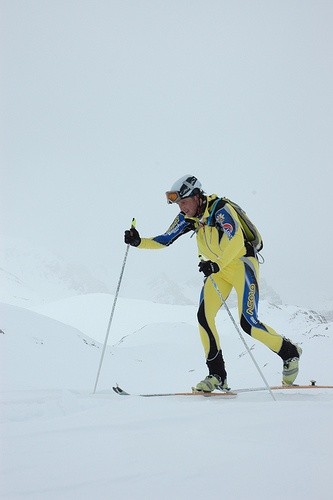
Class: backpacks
208,197,264,264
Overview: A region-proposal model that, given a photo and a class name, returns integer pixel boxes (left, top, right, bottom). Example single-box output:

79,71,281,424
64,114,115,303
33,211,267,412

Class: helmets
169,174,202,204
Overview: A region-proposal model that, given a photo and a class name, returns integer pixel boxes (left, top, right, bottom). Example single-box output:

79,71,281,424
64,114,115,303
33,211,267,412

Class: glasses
164,191,181,204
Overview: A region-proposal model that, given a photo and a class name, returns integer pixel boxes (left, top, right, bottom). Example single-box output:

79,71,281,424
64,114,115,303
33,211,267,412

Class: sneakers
282,345,302,386
194,372,228,392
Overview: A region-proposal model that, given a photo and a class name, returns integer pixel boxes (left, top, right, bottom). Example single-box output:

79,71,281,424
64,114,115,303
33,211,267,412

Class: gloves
124,227,141,247
198,260,220,277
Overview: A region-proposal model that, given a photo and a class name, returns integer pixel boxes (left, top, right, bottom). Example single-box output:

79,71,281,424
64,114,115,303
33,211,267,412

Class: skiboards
112,379,333,397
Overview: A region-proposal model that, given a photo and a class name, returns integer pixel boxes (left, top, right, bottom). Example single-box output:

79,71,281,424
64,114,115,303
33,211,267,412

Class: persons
124,174,302,393
